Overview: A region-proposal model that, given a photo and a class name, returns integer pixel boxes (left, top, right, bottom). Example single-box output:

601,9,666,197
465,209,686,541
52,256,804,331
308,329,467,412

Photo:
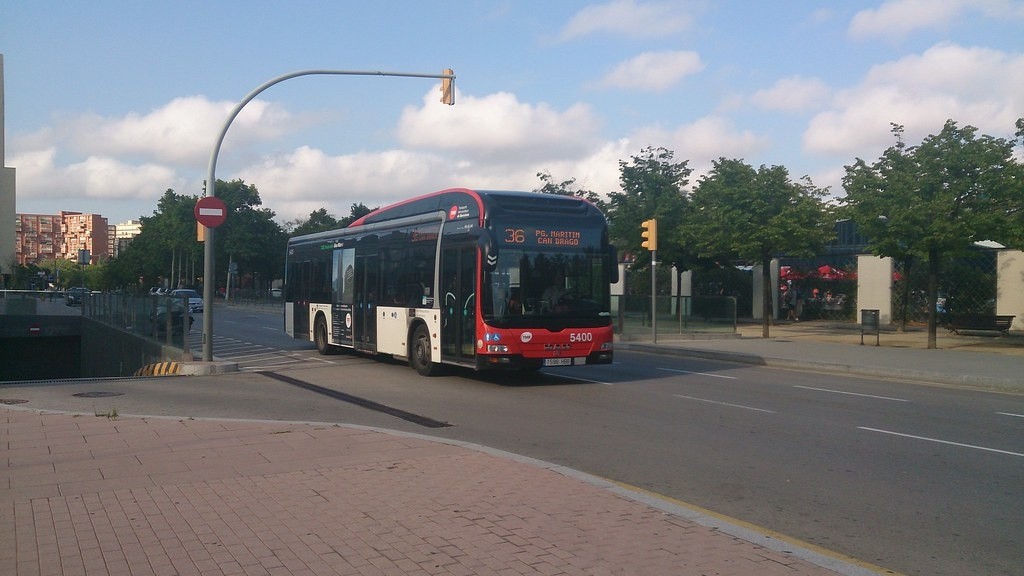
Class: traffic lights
641,219,657,251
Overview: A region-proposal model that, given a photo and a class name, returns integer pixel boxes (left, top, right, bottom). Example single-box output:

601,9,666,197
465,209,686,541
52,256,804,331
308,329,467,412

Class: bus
280,187,620,378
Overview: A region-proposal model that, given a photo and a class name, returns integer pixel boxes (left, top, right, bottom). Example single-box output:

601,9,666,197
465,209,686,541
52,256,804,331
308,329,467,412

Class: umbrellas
807,265,848,279
848,270,904,280
780,266,810,280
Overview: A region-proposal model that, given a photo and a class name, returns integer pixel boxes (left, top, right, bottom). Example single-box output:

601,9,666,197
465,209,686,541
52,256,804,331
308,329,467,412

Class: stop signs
194,197,228,228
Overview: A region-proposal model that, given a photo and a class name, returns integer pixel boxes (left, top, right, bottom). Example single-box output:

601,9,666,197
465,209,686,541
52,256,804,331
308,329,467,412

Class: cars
55,286,194,336
169,289,204,313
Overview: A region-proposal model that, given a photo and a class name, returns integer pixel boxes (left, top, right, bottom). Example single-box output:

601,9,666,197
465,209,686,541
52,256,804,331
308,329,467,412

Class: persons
780,281,800,321
811,288,832,303
219,286,225,296
542,283,563,304
